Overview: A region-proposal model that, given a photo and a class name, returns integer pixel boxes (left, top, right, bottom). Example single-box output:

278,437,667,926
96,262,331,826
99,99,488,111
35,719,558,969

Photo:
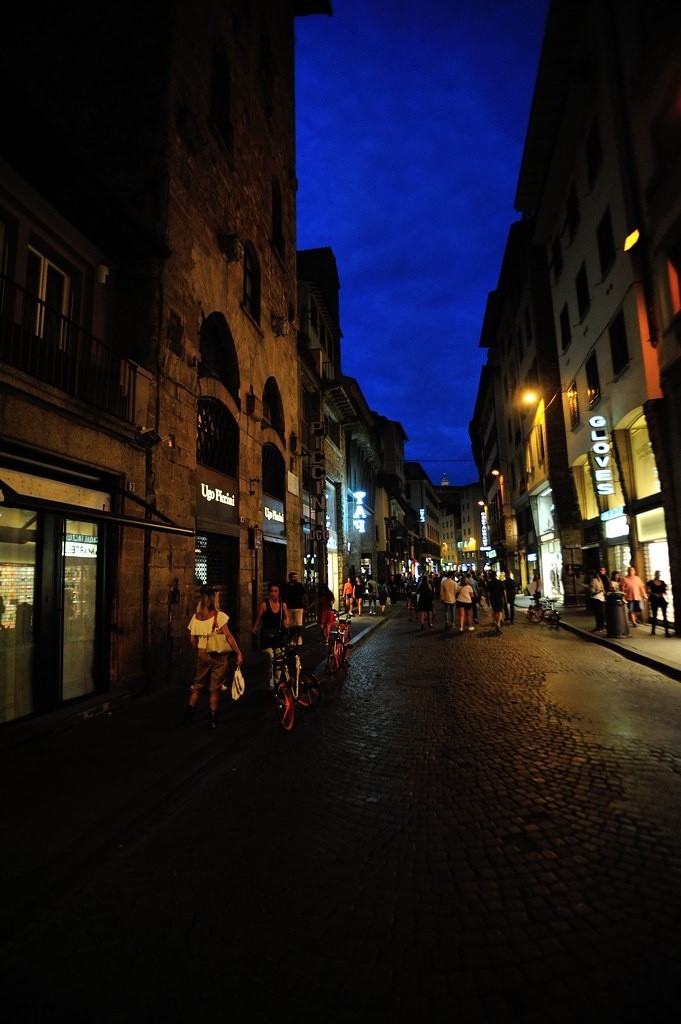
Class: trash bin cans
603,591,630,639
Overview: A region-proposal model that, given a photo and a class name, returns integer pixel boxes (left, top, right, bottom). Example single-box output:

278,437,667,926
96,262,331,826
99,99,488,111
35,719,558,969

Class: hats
200,584,218,593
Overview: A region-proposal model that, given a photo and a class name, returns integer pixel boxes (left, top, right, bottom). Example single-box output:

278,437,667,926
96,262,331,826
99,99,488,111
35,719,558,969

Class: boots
181,705,195,725
208,710,224,729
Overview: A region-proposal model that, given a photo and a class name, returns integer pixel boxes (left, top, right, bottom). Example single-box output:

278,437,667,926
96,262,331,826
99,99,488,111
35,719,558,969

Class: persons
173,539,677,733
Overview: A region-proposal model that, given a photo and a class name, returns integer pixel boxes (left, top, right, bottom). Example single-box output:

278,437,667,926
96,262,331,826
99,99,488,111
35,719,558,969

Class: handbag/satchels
386,596,392,607
206,611,233,654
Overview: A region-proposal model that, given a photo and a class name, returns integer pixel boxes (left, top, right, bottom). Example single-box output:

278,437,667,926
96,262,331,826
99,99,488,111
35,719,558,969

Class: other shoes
468,626,475,630
289,639,302,645
593,625,605,634
421,623,424,630
429,623,433,627
348,610,354,615
651,629,655,635
369,610,377,615
323,634,329,647
459,627,464,632
664,631,673,638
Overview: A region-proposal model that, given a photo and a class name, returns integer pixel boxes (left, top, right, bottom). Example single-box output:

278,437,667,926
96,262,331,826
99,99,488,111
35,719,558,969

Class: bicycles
326,607,353,676
269,642,323,734
541,599,560,629
525,590,544,624
